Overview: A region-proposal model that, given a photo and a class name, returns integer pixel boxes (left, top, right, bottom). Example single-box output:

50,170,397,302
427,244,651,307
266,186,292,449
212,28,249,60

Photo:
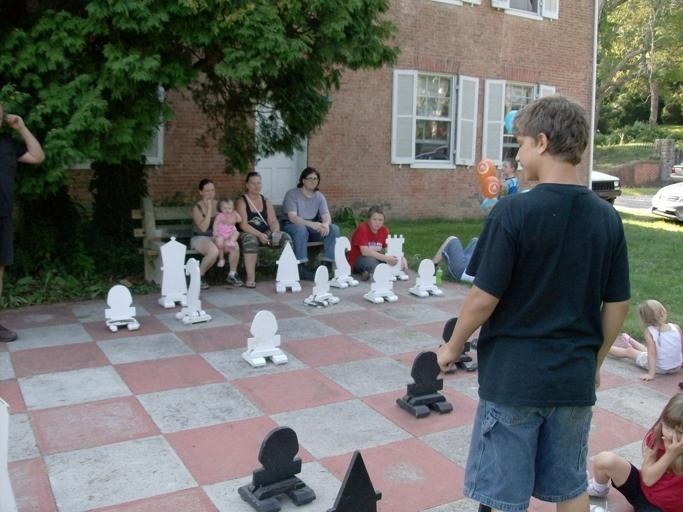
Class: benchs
129,195,334,288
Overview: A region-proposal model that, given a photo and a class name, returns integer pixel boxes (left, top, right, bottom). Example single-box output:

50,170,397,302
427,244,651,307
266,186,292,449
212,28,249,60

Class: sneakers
587,479,609,498
300,271,314,282
201,281,210,290
216,259,226,268
226,242,236,250
245,281,257,288
359,270,370,281
329,271,332,280
225,272,244,287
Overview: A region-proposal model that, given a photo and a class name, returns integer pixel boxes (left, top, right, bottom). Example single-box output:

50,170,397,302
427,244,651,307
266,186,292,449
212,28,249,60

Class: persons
433,236,480,284
586,393,683,512
0,101,45,342
189,167,341,290
497,158,519,200
608,299,683,381
436,95,630,512
346,205,409,281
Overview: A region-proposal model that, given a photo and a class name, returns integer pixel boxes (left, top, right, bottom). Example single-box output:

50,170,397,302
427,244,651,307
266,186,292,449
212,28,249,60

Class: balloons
476,160,500,213
504,110,518,134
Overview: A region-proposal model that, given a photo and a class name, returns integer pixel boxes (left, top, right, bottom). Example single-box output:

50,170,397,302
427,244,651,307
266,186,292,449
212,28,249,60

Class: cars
651,181,683,225
669,161,682,183
591,168,623,209
501,149,522,164
415,144,448,159
